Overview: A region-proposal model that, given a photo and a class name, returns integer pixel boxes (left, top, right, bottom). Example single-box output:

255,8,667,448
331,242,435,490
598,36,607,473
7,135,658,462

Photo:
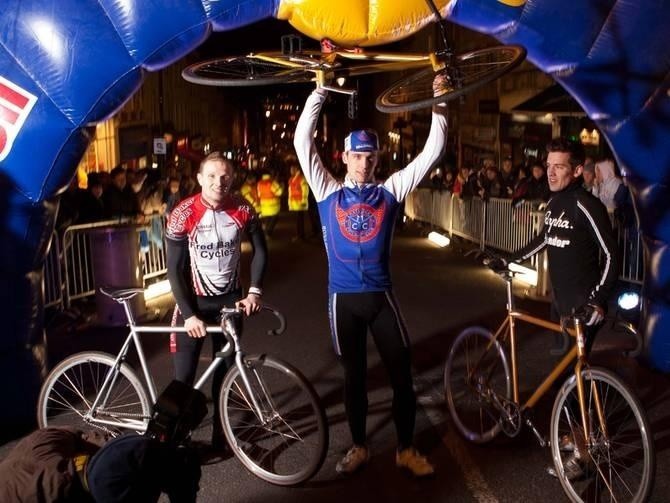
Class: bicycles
36,285,328,486
177,0,527,116
446,266,655,503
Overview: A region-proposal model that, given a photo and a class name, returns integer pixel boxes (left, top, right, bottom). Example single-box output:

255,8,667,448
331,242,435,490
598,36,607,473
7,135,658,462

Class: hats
86,434,165,503
345,129,381,153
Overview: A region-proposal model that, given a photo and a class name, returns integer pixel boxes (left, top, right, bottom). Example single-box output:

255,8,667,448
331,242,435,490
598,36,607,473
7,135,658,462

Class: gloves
489,257,509,274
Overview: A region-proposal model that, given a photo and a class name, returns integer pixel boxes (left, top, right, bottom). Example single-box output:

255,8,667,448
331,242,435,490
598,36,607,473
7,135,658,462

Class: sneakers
211,431,255,456
396,447,435,478
336,447,370,475
264,226,324,245
546,456,587,481
559,434,599,452
177,438,210,449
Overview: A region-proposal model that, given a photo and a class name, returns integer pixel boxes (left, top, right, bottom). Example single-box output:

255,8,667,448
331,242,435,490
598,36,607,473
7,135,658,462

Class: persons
49,135,200,315
230,144,347,242
0,427,201,502
375,153,553,203
577,117,622,214
489,137,623,483
164,149,268,450
292,71,455,481
